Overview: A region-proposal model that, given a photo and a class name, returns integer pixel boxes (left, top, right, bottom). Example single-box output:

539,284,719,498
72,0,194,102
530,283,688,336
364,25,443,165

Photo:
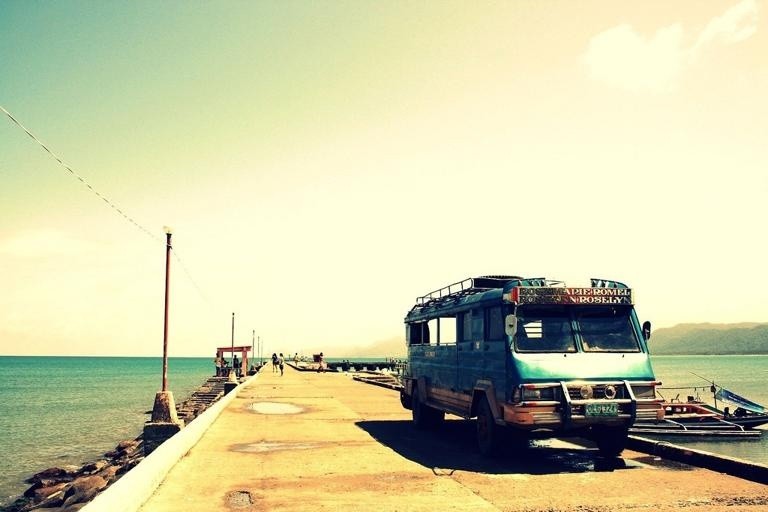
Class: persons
222,361,227,367
689,396,695,403
386,357,401,363
221,358,225,367
233,355,238,368
271,353,279,373
317,352,325,373
214,353,221,376
294,353,298,366
724,407,731,418
278,353,284,376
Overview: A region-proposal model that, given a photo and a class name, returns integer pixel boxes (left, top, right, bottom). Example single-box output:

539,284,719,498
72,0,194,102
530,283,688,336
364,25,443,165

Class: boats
629,386,768,441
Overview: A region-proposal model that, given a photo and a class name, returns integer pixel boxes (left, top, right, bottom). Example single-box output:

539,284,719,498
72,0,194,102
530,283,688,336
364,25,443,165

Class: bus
401,274,665,461
